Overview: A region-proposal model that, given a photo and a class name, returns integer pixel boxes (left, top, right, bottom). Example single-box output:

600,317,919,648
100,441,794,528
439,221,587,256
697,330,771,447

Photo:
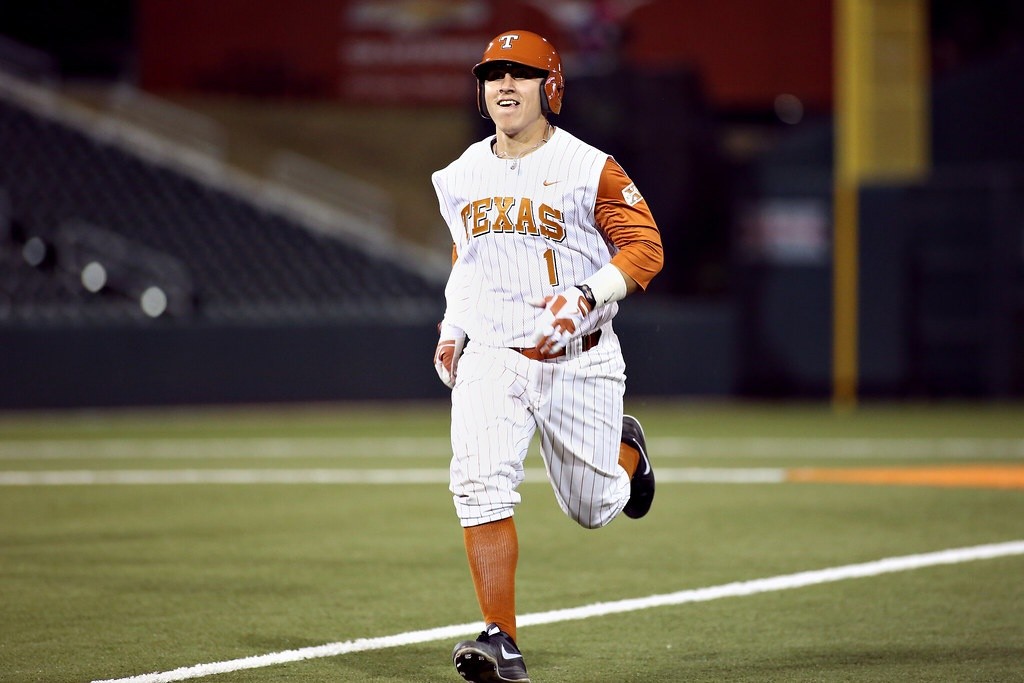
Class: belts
508,328,602,360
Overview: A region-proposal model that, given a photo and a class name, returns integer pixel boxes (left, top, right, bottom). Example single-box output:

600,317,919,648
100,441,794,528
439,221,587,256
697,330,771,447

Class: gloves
434,320,466,389
528,286,592,354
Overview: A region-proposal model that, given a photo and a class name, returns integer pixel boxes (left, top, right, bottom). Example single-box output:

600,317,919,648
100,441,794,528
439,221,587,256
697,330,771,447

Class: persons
431,29,664,682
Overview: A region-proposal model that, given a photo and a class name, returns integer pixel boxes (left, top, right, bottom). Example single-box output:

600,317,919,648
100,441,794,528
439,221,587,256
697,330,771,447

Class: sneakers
452,623,530,683
623,414,656,519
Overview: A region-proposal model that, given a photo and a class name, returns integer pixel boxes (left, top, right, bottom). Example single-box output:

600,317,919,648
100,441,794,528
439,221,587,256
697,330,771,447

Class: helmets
472,29,565,119
